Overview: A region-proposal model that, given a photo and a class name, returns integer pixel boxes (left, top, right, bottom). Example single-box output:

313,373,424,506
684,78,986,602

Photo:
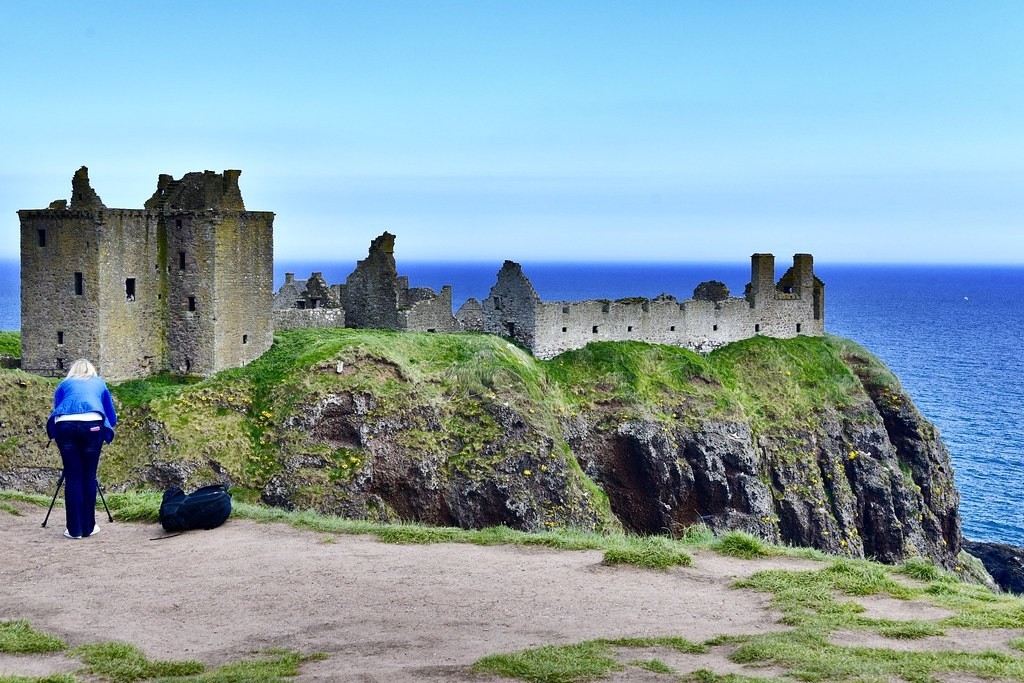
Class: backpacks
158,483,231,533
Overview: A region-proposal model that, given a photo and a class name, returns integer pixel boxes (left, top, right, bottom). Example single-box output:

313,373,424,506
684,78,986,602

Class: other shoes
63,529,82,539
90,524,100,535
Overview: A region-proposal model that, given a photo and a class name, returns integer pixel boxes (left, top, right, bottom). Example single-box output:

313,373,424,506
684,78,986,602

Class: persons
46,358,117,538
